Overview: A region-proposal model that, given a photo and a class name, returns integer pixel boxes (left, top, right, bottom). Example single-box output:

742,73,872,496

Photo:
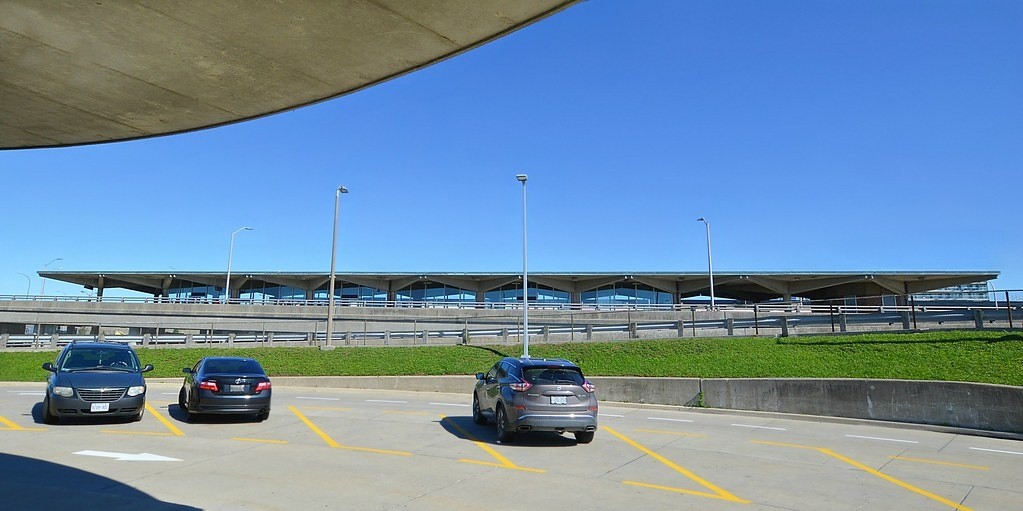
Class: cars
179,357,272,420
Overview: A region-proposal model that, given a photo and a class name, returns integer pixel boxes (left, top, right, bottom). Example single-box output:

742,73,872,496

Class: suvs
471,356,599,444
42,339,154,422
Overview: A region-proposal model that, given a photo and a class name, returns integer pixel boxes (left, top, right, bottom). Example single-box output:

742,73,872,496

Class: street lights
42,258,64,293
18,271,31,300
226,228,255,302
515,173,529,358
327,186,351,345
698,217,715,309
